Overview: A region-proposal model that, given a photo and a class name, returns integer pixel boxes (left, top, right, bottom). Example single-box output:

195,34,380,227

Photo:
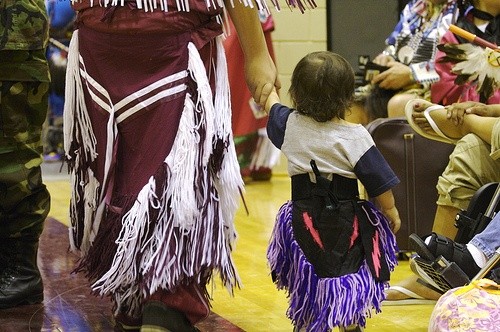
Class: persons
341,0,500,332
264,51,401,330
0,0,280,332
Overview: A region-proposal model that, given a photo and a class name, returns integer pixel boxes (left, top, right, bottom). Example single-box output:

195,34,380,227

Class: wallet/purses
363,61,390,83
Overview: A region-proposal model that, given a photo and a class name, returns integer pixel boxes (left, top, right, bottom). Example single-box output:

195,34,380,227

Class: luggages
365,118,457,260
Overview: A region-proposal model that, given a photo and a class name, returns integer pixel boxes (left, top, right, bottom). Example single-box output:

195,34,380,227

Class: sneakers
410,256,471,292
141,301,201,332
115,320,143,329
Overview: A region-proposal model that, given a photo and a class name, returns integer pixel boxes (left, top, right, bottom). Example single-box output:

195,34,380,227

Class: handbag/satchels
428,279,500,332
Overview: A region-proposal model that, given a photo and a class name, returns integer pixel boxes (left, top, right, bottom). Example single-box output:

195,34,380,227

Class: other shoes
44,153,61,160
252,173,271,180
345,325,362,332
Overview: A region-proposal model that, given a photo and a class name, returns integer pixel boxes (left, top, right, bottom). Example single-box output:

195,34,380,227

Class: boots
0,238,44,309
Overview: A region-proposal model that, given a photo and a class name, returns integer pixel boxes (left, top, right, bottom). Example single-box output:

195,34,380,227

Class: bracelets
408,66,417,84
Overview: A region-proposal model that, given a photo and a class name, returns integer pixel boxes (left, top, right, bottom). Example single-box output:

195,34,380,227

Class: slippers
405,99,460,145
382,286,437,305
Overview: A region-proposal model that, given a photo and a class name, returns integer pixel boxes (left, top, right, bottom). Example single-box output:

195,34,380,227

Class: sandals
409,232,500,287
